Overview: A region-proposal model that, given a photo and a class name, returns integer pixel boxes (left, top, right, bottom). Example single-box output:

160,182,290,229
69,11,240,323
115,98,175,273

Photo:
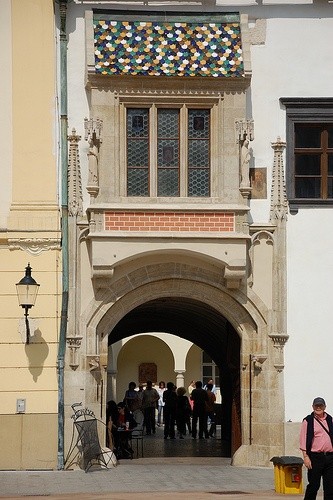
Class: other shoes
170,435,176,439
179,436,184,439
208,432,214,437
164,434,168,439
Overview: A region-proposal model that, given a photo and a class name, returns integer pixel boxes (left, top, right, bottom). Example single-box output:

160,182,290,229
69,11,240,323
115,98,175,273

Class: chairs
130,414,146,458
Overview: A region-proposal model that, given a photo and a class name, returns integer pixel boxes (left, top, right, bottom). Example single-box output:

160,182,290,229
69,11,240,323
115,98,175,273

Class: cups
126,421,129,430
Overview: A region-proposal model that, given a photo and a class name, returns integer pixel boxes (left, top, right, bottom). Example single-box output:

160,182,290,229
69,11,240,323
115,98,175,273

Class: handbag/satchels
185,397,192,417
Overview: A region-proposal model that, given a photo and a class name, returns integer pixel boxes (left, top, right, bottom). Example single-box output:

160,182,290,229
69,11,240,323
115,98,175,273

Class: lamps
16,262,41,345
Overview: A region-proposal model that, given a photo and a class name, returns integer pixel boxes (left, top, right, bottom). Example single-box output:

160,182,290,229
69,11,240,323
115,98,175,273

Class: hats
312,397,325,405
147,380,153,384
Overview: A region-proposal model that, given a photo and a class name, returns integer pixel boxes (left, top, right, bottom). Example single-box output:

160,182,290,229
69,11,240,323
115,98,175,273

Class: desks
115,430,133,459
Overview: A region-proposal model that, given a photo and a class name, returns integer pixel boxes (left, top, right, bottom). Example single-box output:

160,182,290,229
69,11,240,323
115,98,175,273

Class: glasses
314,404,325,407
117,407,122,410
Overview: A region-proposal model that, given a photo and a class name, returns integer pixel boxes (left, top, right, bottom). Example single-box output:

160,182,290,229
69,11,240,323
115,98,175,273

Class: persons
240,140,253,189
106,399,145,458
301,397,332,499
163,382,190,440
125,381,167,436
87,139,99,185
187,377,216,440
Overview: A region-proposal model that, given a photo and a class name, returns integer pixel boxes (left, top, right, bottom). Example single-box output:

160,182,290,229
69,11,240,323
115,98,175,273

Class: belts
312,451,333,455
